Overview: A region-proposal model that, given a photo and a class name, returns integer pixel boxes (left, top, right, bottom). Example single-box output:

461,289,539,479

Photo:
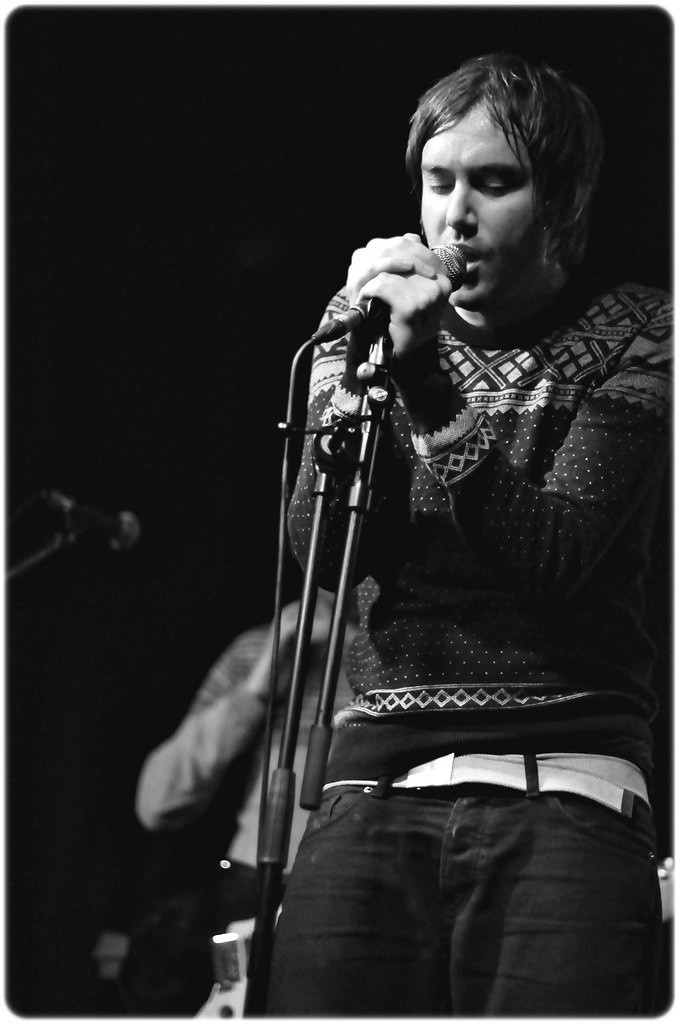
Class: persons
137,582,341,1017
264,46,674,1021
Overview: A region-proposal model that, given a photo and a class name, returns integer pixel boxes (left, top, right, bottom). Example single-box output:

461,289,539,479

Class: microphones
310,242,465,345
